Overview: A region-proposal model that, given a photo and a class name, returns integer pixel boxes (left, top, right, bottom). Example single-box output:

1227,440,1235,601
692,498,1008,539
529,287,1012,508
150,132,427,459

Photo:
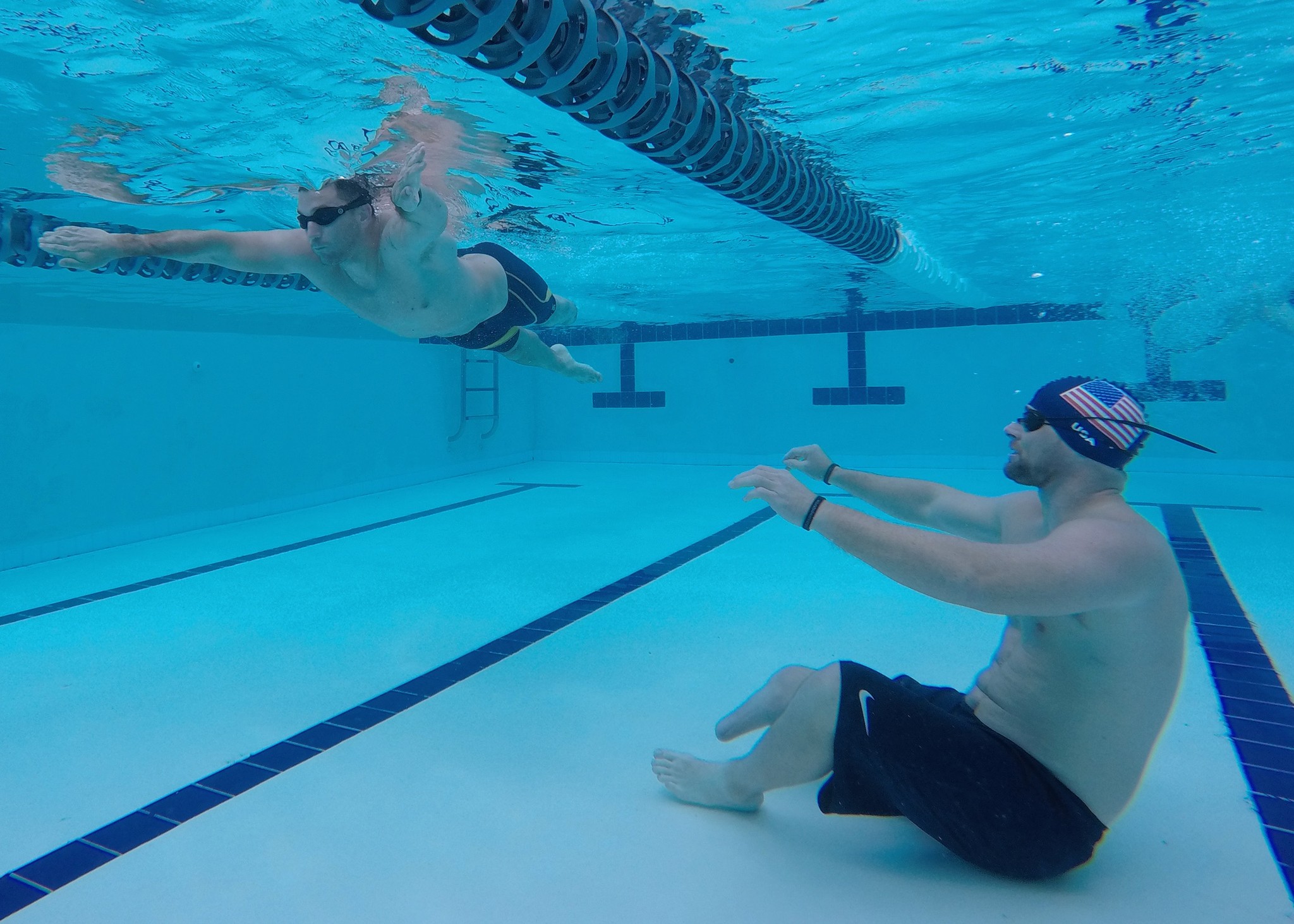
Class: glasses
296,194,370,229
1018,410,1134,460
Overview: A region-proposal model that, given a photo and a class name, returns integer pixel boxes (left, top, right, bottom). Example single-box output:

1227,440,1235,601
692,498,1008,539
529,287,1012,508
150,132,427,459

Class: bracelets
802,495,827,531
823,463,840,485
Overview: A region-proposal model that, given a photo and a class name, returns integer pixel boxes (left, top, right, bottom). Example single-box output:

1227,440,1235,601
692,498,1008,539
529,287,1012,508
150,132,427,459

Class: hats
1027,375,1149,473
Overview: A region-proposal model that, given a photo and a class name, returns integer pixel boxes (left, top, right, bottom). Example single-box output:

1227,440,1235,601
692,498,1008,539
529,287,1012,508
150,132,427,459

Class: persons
652,375,1189,881
36,142,602,386
